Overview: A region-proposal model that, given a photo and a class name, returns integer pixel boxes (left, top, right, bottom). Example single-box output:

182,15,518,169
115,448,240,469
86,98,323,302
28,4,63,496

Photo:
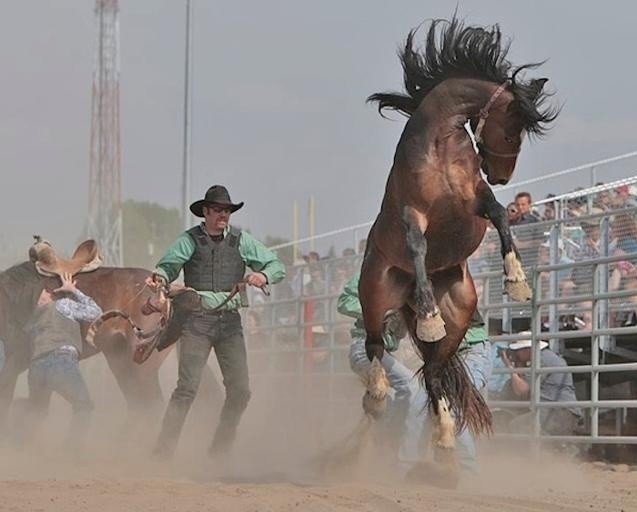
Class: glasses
202,204,232,215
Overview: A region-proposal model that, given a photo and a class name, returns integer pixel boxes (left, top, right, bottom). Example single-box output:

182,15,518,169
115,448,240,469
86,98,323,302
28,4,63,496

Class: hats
190,183,245,218
21,281,43,306
573,208,605,225
508,328,548,355
540,238,564,249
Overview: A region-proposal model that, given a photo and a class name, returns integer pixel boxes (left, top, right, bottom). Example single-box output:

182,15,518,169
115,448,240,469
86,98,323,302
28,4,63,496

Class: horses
357,18,555,465
0,261,176,426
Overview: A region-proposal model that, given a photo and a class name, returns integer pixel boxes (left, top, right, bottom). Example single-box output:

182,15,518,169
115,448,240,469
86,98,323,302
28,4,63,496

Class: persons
338,271,430,469
471,183,637,338
144,185,287,472
496,331,583,463
244,238,370,346
447,309,493,492
23,271,104,418
